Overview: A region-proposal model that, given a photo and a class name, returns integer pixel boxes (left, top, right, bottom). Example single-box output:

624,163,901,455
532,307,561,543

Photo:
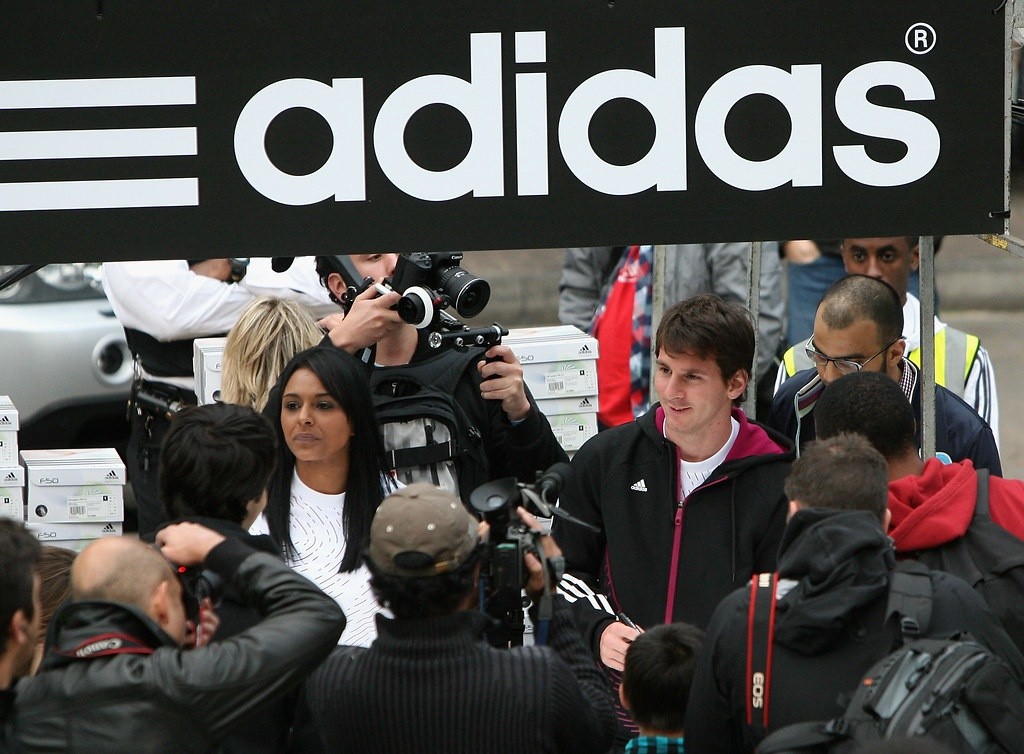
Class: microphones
539,462,576,496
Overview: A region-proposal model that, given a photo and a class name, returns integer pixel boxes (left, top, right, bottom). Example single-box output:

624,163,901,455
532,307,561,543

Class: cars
0,264,136,451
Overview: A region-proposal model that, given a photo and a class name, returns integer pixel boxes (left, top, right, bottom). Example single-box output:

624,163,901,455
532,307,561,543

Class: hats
369,483,477,578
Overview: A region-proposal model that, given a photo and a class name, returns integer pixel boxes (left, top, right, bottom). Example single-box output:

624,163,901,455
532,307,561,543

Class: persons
548,293,797,749
1,236,1023,753
10,521,346,752
305,485,622,753
141,402,285,568
247,346,405,652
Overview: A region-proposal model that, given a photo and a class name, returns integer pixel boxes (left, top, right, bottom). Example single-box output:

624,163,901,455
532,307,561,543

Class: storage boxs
193,337,227,407
535,394,599,451
0,395,21,468
500,324,600,400
19,448,127,524
23,505,123,554
0,466,26,523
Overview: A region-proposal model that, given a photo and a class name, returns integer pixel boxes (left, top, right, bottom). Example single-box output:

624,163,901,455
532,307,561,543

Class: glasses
805,328,901,375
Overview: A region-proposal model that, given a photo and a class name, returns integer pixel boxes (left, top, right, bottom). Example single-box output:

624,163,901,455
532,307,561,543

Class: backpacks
754,560,1024,754
914,467,1024,647
361,347,487,511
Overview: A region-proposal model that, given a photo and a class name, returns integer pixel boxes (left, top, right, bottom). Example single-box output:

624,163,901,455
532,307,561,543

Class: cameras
152,545,226,624
391,252,491,318
